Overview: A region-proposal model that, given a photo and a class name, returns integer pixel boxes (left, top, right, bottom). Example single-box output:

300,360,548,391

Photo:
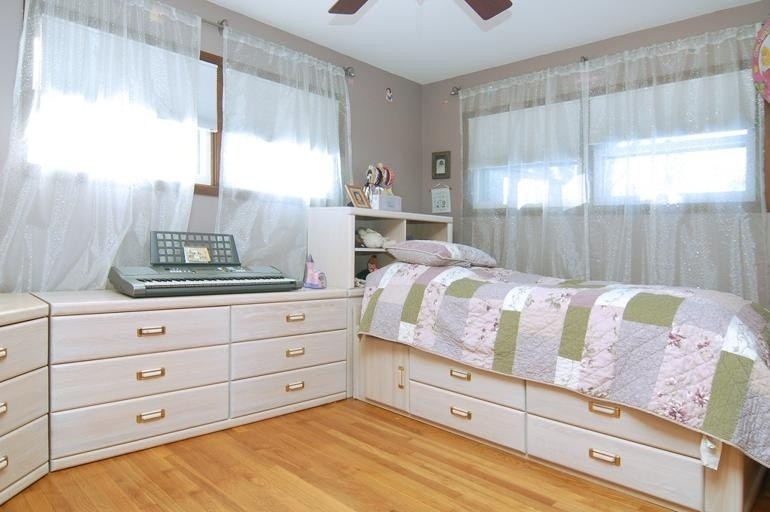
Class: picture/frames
432,150,451,180
345,183,371,209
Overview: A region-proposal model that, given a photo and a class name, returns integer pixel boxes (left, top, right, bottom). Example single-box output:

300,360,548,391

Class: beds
353,261,770,512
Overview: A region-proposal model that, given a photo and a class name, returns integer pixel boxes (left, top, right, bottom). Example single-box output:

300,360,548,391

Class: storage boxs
368,194,402,211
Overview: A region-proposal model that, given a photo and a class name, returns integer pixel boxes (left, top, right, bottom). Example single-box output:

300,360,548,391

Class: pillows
384,240,497,267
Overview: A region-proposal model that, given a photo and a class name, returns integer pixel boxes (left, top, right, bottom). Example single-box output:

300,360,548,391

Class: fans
328,0,512,21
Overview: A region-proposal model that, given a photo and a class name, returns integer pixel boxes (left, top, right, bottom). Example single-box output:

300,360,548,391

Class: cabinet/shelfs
364,332,408,413
0,292,50,506
526,380,704,512
408,347,526,455
307,206,454,289
229,286,354,430
29,290,230,474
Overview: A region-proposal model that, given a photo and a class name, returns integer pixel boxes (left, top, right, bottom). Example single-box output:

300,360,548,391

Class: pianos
108,231,304,297
